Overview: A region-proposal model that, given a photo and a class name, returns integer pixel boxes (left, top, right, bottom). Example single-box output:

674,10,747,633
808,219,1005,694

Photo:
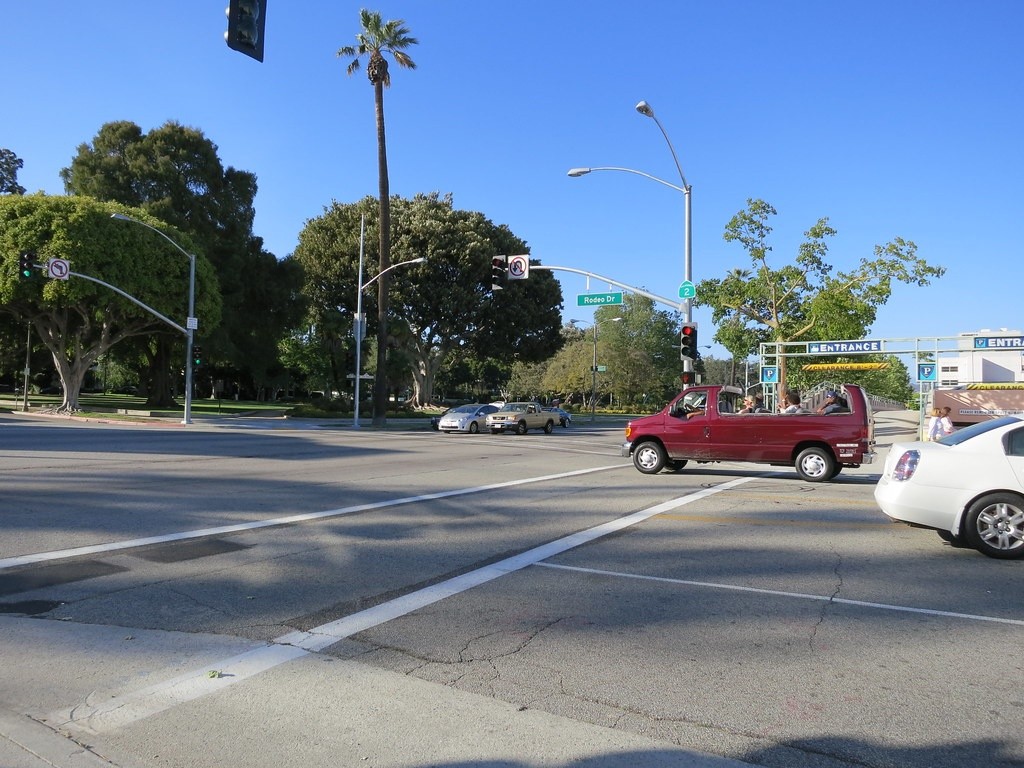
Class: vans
622,384,876,482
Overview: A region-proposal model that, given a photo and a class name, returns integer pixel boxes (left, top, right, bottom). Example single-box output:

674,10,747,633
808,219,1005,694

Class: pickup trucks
486,402,560,435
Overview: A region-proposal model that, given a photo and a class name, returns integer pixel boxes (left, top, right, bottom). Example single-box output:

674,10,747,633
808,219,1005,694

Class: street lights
568,101,693,408
570,317,622,426
354,211,425,427
111,214,195,424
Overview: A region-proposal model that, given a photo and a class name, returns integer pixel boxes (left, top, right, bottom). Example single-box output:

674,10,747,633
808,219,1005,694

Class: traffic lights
682,371,694,384
681,323,696,359
491,255,507,290
19,250,33,282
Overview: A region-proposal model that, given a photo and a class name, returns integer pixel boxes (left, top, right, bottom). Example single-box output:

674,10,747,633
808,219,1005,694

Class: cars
431,401,523,434
874,414,1024,560
541,407,571,428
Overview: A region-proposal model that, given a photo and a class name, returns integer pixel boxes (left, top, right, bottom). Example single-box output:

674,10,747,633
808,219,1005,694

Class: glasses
826,396,832,398
744,399,748,401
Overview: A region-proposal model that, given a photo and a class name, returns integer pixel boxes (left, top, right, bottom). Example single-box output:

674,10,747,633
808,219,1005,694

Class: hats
826,391,837,398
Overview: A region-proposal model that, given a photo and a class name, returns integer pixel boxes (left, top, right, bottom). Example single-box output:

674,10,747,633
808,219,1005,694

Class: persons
685,403,705,419
816,390,848,413
528,407,532,413
941,407,957,434
737,394,756,414
753,393,765,411
777,393,801,414
232,383,239,400
928,408,945,442
